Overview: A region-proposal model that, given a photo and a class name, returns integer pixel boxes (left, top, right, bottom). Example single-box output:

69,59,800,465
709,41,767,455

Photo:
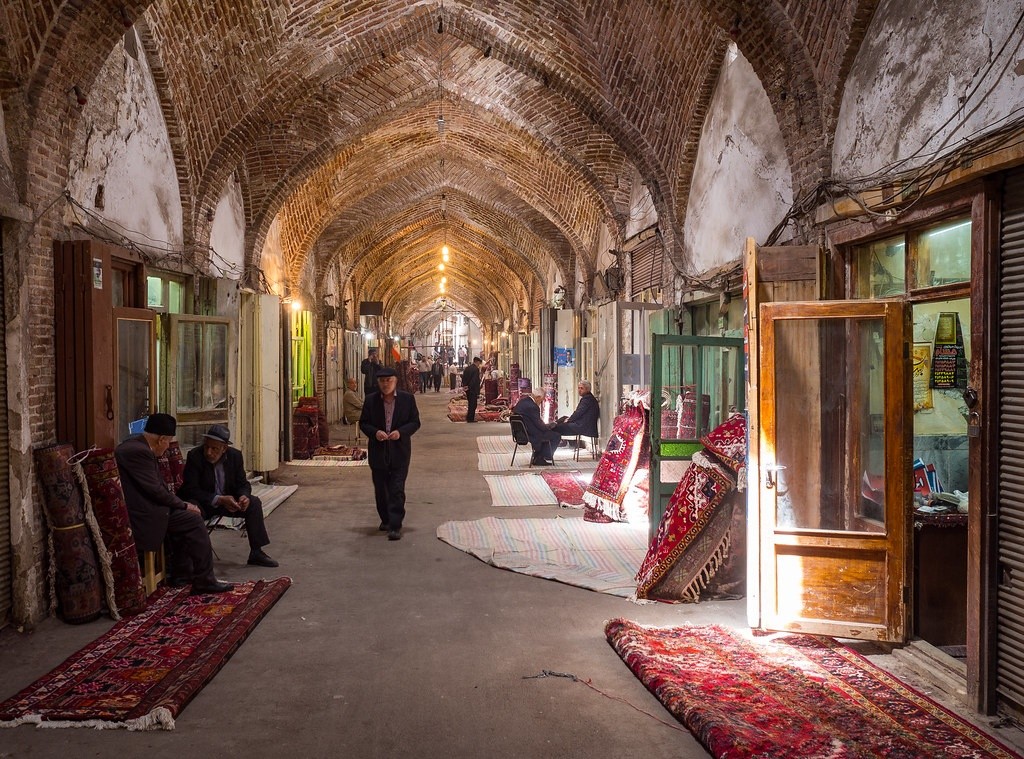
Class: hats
201,425,233,445
474,357,482,362
375,367,398,378
144,413,176,437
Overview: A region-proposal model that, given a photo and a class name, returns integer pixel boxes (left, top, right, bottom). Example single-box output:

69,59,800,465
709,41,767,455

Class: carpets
32,441,186,620
605,617,1024,759
393,360,421,393
447,351,558,425
540,388,711,523
0,575,293,732
634,412,748,602
292,397,367,461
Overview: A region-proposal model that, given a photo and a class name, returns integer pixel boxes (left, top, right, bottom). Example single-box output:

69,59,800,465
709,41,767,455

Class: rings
381,439,383,441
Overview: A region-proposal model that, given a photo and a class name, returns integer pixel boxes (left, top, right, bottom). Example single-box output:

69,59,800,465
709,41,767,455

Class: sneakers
247,550,279,567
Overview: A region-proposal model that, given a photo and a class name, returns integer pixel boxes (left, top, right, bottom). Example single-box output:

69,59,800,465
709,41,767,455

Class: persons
416,354,444,393
361,349,383,396
552,380,599,439
449,362,458,390
435,342,467,366
115,413,233,594
174,424,280,568
512,388,561,467
343,378,364,424
358,367,421,540
462,357,482,422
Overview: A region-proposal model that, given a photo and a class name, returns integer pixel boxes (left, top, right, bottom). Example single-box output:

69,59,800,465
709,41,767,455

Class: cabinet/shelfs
864,498,968,646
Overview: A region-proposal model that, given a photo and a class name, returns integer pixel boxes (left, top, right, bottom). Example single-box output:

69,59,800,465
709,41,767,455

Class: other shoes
379,521,389,530
170,571,204,584
531,456,553,465
467,419,478,423
192,578,234,593
388,527,401,539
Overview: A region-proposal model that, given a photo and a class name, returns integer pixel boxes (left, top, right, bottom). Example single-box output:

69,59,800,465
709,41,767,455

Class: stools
203,513,224,561
139,541,167,597
